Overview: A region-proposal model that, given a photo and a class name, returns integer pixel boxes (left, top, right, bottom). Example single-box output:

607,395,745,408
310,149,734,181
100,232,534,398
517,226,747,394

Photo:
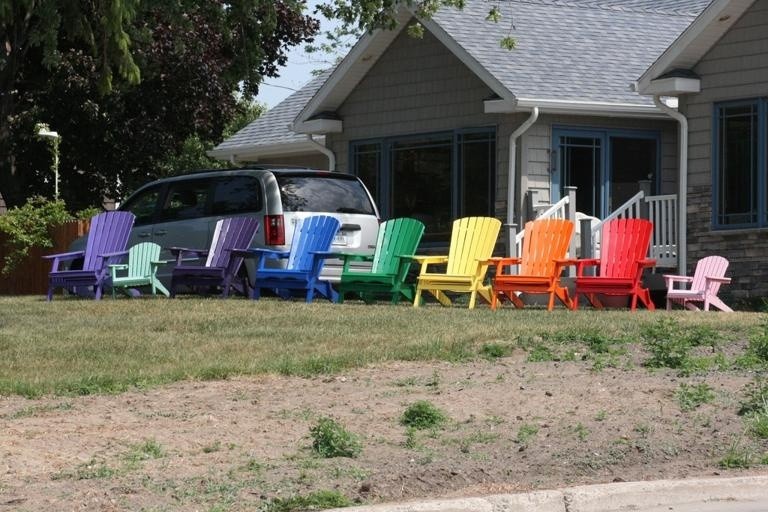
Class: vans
66,165,386,286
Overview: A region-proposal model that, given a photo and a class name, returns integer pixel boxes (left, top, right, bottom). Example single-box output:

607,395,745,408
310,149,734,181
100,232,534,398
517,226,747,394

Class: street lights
40,130,61,202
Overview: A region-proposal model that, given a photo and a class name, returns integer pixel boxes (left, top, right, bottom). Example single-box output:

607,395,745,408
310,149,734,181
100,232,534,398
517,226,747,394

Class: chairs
566,218,658,311
245,214,342,303
163,217,261,298
487,217,574,313
332,216,427,306
105,241,171,300
39,210,142,302
661,254,736,312
414,215,502,311
